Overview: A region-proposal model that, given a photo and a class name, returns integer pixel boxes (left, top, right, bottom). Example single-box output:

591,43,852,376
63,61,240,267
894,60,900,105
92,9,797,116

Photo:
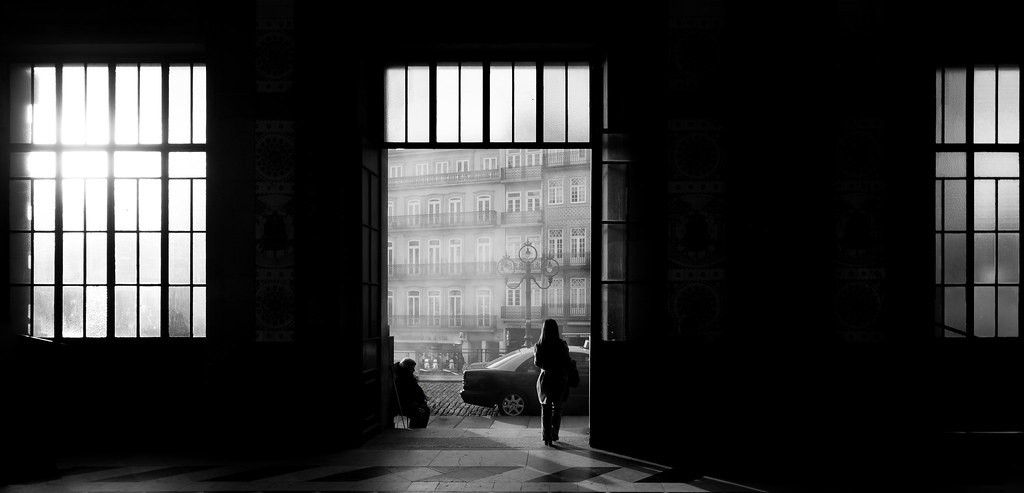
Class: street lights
497,237,559,349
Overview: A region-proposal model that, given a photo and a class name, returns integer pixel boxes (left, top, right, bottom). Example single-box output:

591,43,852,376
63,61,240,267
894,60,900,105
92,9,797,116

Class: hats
403,359,416,365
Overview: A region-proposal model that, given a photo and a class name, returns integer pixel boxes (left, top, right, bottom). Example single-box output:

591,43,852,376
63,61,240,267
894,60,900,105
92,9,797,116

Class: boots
543,425,559,445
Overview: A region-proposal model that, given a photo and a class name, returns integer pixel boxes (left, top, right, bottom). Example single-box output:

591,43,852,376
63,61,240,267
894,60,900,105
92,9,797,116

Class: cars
459,339,590,418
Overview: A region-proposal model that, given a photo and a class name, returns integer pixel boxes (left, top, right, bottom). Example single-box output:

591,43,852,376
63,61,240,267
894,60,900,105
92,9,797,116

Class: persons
535,319,577,445
388,358,430,430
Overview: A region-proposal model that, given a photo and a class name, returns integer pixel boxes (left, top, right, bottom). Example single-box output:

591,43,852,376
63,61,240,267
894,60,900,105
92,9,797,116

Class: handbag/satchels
569,360,580,388
